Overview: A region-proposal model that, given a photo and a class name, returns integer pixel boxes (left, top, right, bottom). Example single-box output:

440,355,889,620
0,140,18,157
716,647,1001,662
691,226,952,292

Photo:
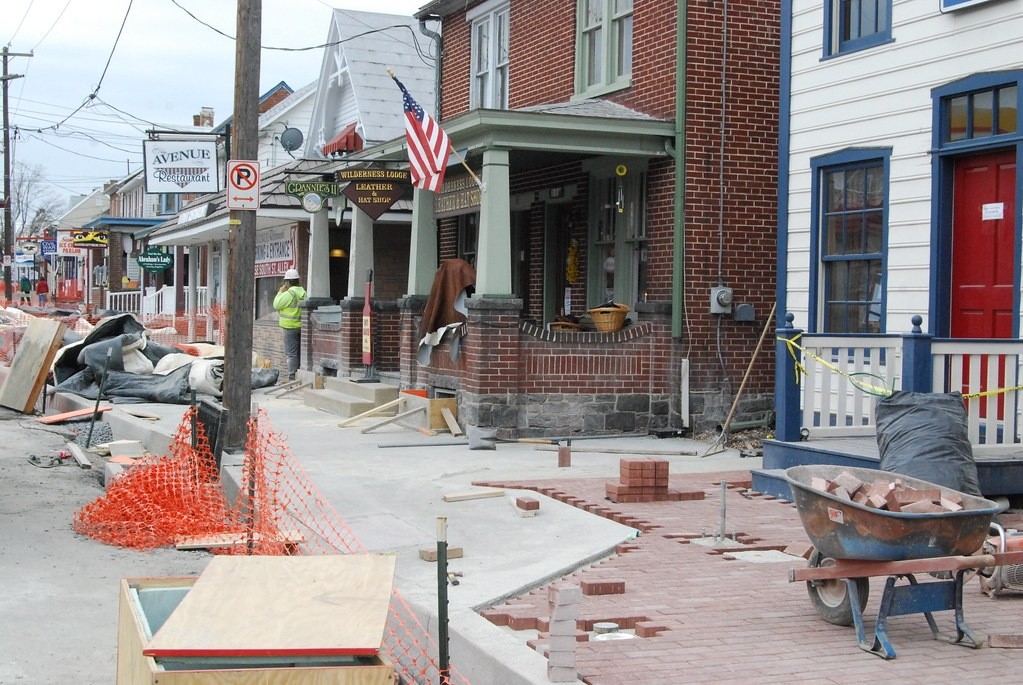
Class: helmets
284,268,300,279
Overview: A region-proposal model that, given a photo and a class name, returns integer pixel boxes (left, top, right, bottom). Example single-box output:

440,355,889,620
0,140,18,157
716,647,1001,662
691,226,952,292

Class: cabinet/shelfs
117,577,396,685
398,391,456,429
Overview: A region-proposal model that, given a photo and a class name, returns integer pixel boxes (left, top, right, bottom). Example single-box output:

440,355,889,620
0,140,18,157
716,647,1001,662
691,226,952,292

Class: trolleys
783,465,1003,660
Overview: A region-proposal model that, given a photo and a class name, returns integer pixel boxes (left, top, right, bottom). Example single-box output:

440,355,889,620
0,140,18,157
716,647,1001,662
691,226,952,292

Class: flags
394,78,451,192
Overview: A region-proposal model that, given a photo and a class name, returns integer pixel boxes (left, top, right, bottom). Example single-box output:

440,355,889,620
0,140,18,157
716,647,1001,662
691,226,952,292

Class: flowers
564,238,579,283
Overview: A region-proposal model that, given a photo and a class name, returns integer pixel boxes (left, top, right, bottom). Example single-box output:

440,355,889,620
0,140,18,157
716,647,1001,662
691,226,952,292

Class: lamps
329,249,348,258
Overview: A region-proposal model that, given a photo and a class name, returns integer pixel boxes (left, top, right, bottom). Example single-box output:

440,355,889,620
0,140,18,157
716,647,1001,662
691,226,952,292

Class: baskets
547,322,584,333
587,302,632,333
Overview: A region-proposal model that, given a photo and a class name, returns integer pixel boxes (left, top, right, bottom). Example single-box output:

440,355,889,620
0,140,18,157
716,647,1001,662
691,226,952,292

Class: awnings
322,123,362,155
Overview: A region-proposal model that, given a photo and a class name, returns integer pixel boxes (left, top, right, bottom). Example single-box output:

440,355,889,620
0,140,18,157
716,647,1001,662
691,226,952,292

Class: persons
20,275,31,306
273,269,306,379
36,277,48,307
